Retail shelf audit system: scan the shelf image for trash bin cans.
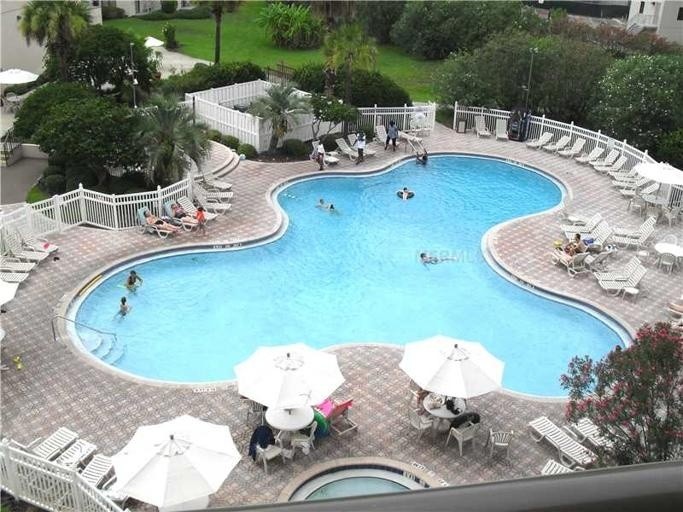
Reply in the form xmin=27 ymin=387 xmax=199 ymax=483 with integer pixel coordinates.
xmin=456 ymin=118 xmax=467 ymax=133
xmin=86 ymin=0 xmax=103 ymax=26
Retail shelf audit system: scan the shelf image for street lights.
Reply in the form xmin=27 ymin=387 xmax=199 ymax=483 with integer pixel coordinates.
xmin=524 ymin=47 xmax=539 ymax=111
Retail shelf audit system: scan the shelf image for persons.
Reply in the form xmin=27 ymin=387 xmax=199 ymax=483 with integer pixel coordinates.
xmin=125 ymin=270 xmax=144 ymax=296
xmin=192 ymin=206 xmax=209 ymax=235
xmin=314 ymin=137 xmax=326 ymax=170
xmin=354 ymin=129 xmax=367 ymax=164
xmin=143 ymin=209 xmax=178 ymax=233
xmin=169 ymin=202 xmax=197 ymax=224
xmin=383 ymin=121 xmax=399 ymax=151
xmin=421 ymin=253 xmax=458 ymax=270
xmin=316 ymin=199 xmax=337 ymax=212
xmin=114 ymin=297 xmax=132 ymax=320
xmin=398 ymin=186 xmax=415 ymax=200
xmin=569 ymin=234 xmax=587 ymax=257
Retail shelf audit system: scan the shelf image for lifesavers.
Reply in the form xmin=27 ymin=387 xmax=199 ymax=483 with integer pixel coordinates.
xmin=397 ymin=191 xmax=414 ymax=198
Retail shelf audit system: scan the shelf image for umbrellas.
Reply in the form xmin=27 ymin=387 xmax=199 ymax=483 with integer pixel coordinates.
xmin=109 ymin=413 xmax=242 ymax=508
xmin=233 ymin=342 xmax=348 ymax=413
xmin=144 ymin=35 xmax=168 ymax=50
xmin=633 ymin=159 xmax=683 ymax=201
xmin=0 ymin=68 xmax=41 ymax=86
xmin=399 ymin=335 xmax=509 ymax=402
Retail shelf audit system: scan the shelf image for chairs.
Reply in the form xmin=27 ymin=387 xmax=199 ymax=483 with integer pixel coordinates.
xmin=473 ymin=114 xmax=509 ymax=142
xmin=0 ymin=218 xmax=59 ymax=284
xmin=311 ymin=125 xmax=423 ymax=169
xmin=9 ymin=425 xmax=129 ymax=509
xmin=239 ymin=396 xmax=359 ymax=473
xmin=135 ymin=165 xmax=233 ymax=240
xmin=408 ymin=380 xmax=607 ymax=478
xmin=527 ymin=132 xmax=683 ymax=303
